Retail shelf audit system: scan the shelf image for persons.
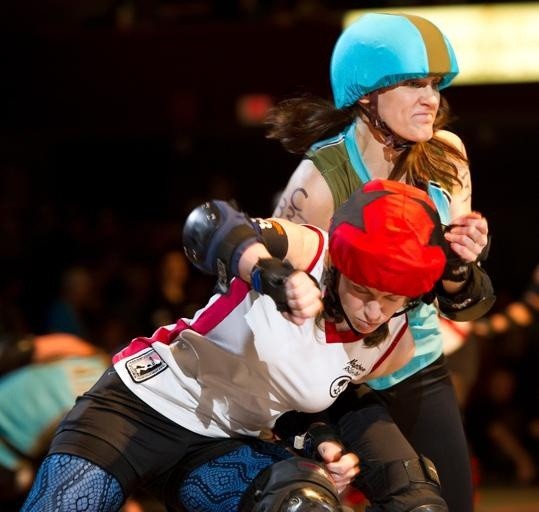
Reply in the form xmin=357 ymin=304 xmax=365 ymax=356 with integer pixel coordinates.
xmin=18 ymin=178 xmax=448 ymax=511
xmin=454 ymin=286 xmax=539 ymax=483
xmin=1 ymin=164 xmax=249 ymax=511
xmin=261 ymin=12 xmax=500 ymax=512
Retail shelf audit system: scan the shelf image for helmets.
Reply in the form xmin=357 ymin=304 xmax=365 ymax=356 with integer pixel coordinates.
xmin=328 ymin=179 xmax=448 ymax=298
xmin=329 ymin=13 xmax=459 ymax=113
